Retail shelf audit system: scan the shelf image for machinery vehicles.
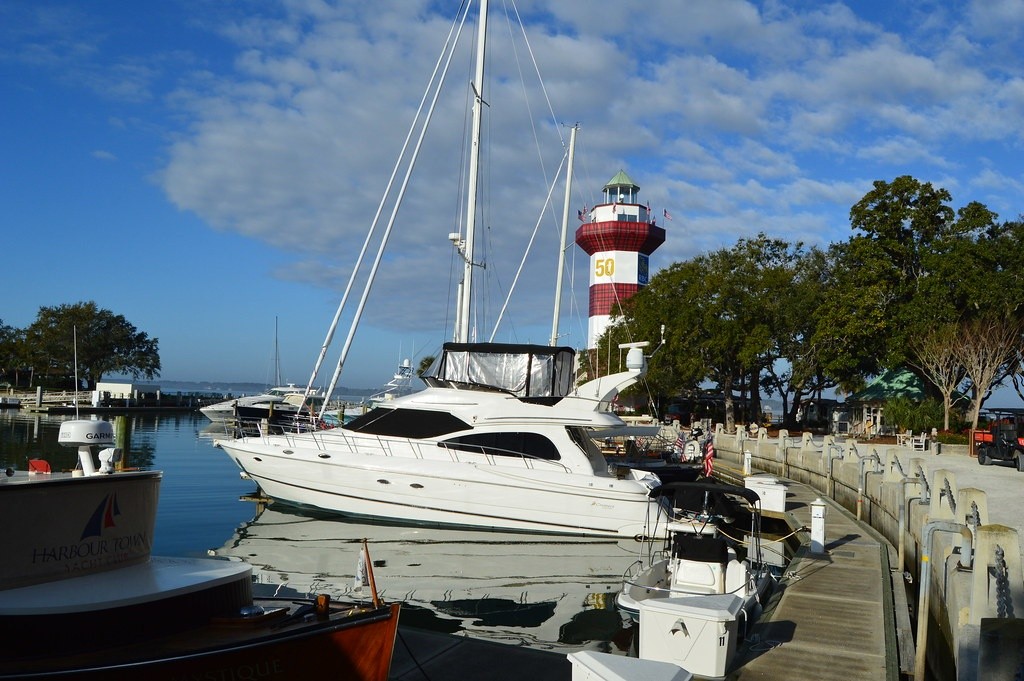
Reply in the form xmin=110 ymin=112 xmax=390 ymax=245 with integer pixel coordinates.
xmin=972 ymin=406 xmax=1024 ymax=471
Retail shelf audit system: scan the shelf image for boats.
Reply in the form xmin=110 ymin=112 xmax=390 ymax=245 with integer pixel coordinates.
xmin=598 ymin=480 xmax=792 ymax=631
xmin=0 ymin=405 xmax=400 ymax=679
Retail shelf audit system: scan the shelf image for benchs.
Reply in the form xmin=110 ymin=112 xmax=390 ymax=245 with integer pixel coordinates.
xmin=693 ymin=418 xmax=712 ymax=432
xmin=910 ymin=432 xmax=927 ymax=452
xmin=896 ymin=429 xmax=912 ymax=446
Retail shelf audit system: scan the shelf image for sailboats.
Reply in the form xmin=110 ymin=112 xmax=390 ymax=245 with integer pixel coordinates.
xmin=197 ymin=0 xmax=738 ymax=538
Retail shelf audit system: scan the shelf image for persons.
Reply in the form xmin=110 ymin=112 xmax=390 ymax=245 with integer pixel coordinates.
xmin=832 ymin=407 xmax=847 ymax=436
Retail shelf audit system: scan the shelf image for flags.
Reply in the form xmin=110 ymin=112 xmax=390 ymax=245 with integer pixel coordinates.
xmin=577 ymin=208 xmax=586 ymax=224
xmin=612 ymin=200 xmax=616 ymax=214
xmin=702 ymin=431 xmax=714 ymax=478
xmin=675 ymin=431 xmax=689 ymax=463
xmin=582 ymin=203 xmax=588 ymax=216
xmin=663 ymin=208 xmax=672 ymax=221
xmin=647 ymin=203 xmax=650 ymax=216
